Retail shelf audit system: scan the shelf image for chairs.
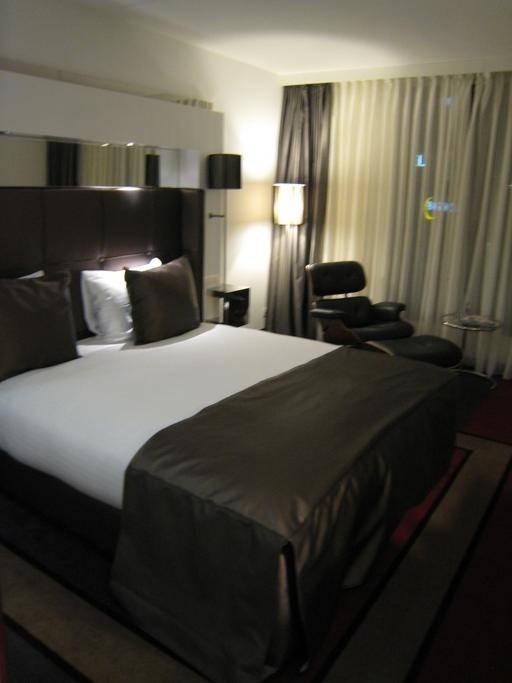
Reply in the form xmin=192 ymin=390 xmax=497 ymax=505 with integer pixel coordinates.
xmin=305 ymin=260 xmax=463 ymax=371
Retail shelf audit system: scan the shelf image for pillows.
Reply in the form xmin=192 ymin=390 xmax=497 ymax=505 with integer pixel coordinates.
xmin=0 ymin=271 xmax=83 ymax=383
xmin=124 ymin=254 xmax=202 ymax=345
xmin=16 ymin=267 xmax=45 ymax=277
xmin=80 ymin=256 xmax=164 ymax=339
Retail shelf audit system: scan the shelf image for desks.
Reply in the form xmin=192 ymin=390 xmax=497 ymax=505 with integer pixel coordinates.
xmin=439 ymin=312 xmax=502 ymax=404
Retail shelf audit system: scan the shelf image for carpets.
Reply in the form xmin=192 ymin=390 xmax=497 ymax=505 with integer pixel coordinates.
xmin=1 ymin=429 xmax=511 ymax=683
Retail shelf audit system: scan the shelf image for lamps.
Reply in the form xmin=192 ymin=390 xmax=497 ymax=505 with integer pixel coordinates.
xmin=272 ymin=183 xmax=305 ymax=226
xmin=1 ymin=185 xmax=460 ymax=683
xmin=205 ymin=154 xmax=242 ymax=323
